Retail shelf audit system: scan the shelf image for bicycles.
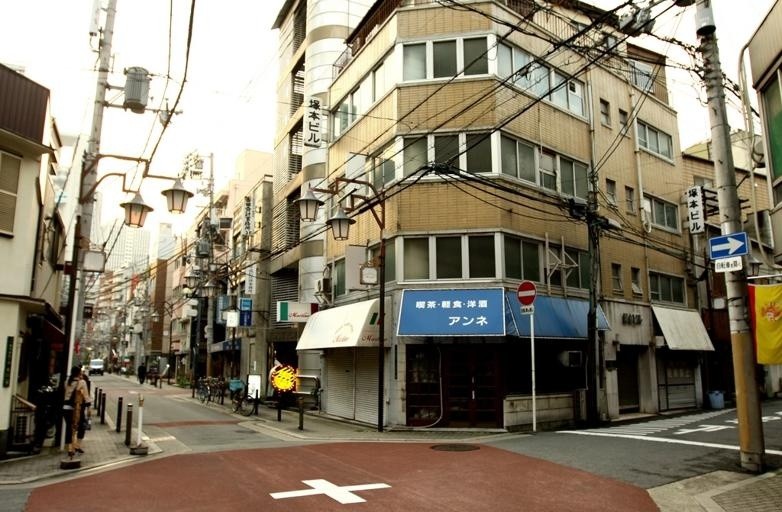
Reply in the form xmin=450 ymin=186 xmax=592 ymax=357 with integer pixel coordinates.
xmin=198 ymin=376 xmax=255 ymax=417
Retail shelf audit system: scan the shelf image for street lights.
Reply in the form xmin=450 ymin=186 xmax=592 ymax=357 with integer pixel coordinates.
xmin=53 ymin=151 xmax=195 ymax=453
xmin=292 ymin=176 xmax=386 ymax=432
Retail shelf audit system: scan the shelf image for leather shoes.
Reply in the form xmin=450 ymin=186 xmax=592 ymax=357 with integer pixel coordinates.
xmin=68 ymin=451 xmax=75 ymax=458
xmin=74 ymin=448 xmax=83 ymax=454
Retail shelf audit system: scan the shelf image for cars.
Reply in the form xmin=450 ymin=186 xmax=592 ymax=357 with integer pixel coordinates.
xmin=88 ymin=359 xmax=104 ymax=376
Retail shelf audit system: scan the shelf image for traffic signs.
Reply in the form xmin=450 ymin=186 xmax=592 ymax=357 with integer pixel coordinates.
xmin=518 ymin=280 xmax=538 ymax=306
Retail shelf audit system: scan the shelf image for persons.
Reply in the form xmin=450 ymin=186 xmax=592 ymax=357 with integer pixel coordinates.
xmin=138 ymin=362 xmax=147 ymax=384
xmin=63 ymin=364 xmax=92 ymax=458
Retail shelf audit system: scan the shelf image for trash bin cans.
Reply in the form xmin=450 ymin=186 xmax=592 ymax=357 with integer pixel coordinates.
xmin=574 ymin=389 xmax=594 ymax=428
xmin=11 ymin=407 xmax=35 ymax=451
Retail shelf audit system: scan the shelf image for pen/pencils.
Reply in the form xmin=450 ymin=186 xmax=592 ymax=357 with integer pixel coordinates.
xmin=708 ymin=391 xmax=725 ymax=409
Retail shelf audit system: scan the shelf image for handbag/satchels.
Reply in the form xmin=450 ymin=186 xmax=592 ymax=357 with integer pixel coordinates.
xmin=81 ymin=417 xmax=92 ymax=429
xmin=68 ymin=390 xmax=86 ymax=413
